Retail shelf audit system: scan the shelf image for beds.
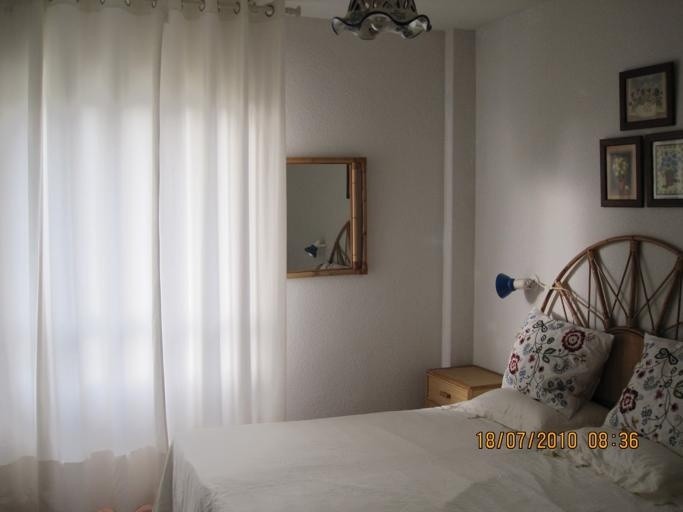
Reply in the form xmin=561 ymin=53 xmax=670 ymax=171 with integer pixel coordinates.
xmin=150 ymin=387 xmax=682 ymax=511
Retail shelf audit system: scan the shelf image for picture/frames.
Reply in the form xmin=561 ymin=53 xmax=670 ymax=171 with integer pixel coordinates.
xmin=598 ymin=60 xmax=683 ymax=207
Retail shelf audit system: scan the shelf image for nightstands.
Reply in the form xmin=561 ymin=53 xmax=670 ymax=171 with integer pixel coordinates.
xmin=423 ymin=365 xmax=504 ymax=410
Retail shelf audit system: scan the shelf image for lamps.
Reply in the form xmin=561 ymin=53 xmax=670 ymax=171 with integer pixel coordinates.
xmin=495 ymin=273 xmax=537 ymax=299
xmin=329 ymin=1 xmax=432 ymax=40
xmin=303 ymin=239 xmax=327 ymax=259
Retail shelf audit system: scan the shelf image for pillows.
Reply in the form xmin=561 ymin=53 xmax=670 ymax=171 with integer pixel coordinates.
xmin=502 ymin=307 xmax=616 ymax=420
xmin=457 ymin=386 xmax=610 ymax=441
xmin=601 ymin=332 xmax=682 ymax=456
xmin=538 ymin=427 xmax=682 ymax=504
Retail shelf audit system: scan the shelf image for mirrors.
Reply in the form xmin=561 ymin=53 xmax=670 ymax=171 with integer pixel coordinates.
xmin=284 ymin=157 xmax=368 ymax=279
